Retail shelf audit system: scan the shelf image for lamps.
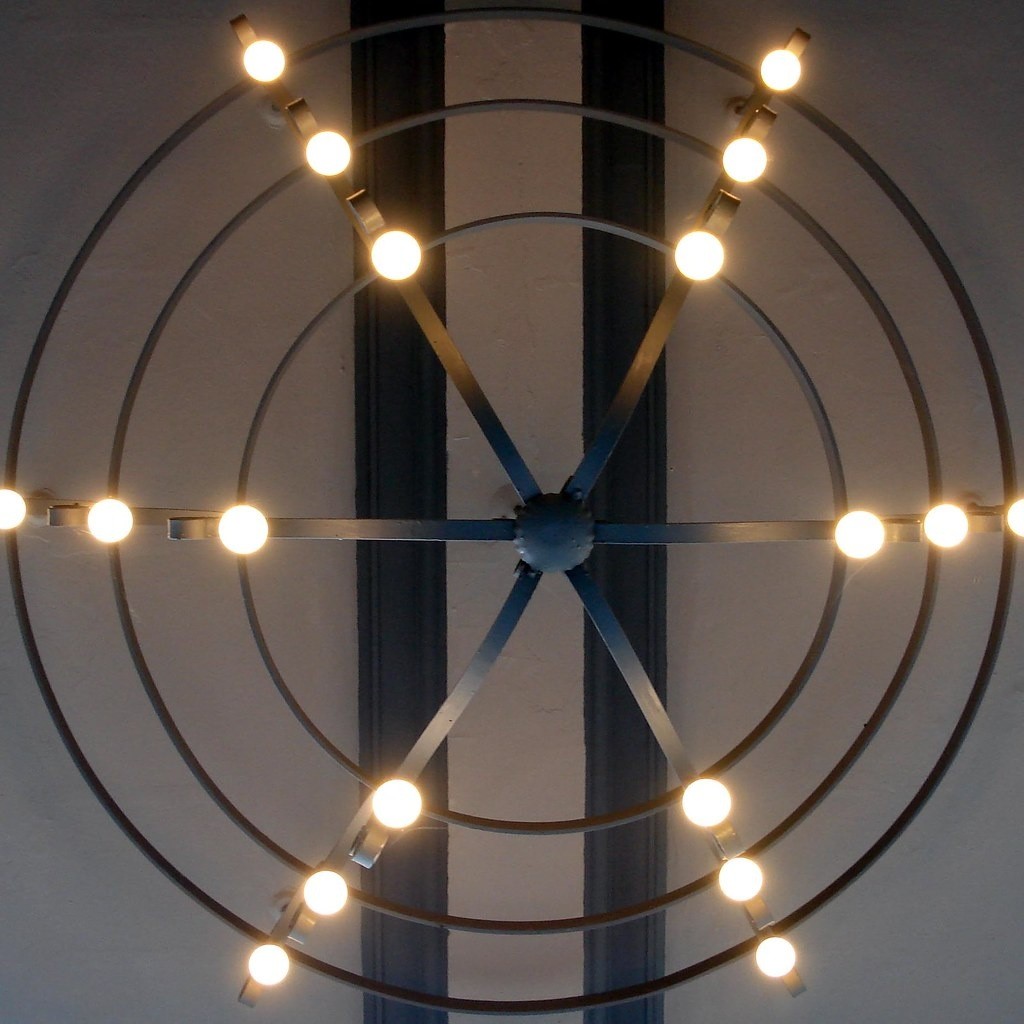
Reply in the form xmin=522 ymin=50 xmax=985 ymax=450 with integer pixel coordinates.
xmin=0 ymin=10 xmax=1024 ymax=1015
